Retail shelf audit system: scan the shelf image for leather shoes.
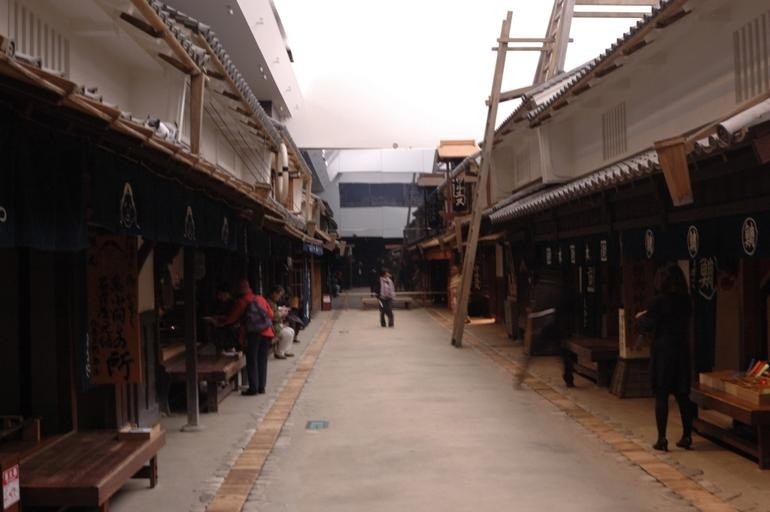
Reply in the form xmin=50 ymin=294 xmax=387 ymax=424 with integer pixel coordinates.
xmin=242 ymin=390 xmax=258 ymax=395
xmin=259 ymin=388 xmax=264 ymax=393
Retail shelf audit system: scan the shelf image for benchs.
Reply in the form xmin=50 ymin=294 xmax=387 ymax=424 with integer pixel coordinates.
xmin=559 ymin=335 xmax=618 ymax=387
xmin=165 ymin=352 xmax=247 ymax=414
xmin=20 ymin=428 xmax=166 ymax=511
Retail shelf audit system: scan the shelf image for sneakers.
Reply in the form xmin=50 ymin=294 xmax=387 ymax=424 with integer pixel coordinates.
xmin=275 ymin=353 xmax=286 ymax=359
xmin=564 ymin=375 xmax=575 ymax=387
xmin=285 ymin=353 xmax=294 ymax=356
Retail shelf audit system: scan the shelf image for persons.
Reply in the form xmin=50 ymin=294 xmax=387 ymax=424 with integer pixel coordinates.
xmin=635 ymin=268 xmax=696 ymax=452
xmin=375 ymin=268 xmax=396 ymax=327
xmin=366 ymin=265 xmax=378 ymax=296
xmin=205 ymin=275 xmax=302 ymax=397
xmin=334 ymin=272 xmax=345 ymax=296
xmin=448 ymin=265 xmax=472 ymax=324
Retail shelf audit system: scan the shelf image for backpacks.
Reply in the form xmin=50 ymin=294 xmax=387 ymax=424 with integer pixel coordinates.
xmin=240 ymin=294 xmax=273 ymax=334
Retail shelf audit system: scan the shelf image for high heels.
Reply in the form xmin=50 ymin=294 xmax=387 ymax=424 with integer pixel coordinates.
xmin=654 ymin=437 xmax=667 ymax=451
xmin=677 ymin=435 xmax=691 ymax=449
